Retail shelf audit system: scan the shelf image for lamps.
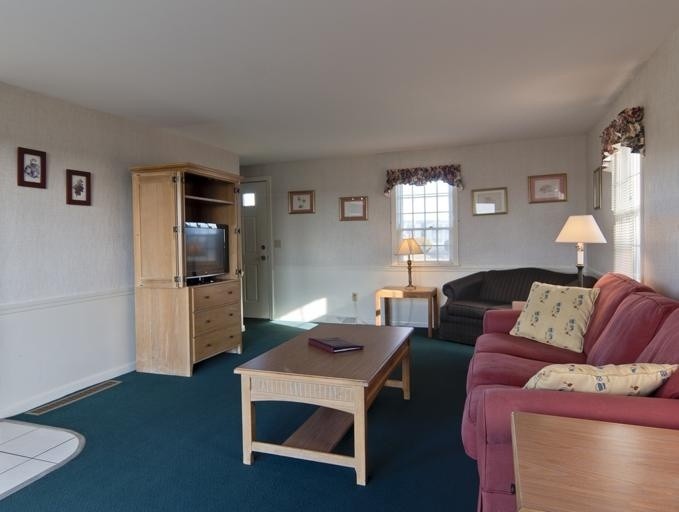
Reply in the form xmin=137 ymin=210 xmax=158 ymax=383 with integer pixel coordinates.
xmin=553 ymin=214 xmax=608 ymax=287
xmin=394 ymin=238 xmax=424 ymax=290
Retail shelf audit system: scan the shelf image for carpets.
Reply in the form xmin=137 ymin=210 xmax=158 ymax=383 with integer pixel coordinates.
xmin=0 ymin=418 xmax=87 ymax=505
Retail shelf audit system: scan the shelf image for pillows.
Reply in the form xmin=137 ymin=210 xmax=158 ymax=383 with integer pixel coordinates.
xmin=508 ymin=281 xmax=600 ymax=353
xmin=522 ymin=363 xmax=679 ymax=396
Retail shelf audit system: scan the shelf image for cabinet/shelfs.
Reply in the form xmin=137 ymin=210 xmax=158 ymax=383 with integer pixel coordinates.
xmin=128 ymin=162 xmax=244 ymax=378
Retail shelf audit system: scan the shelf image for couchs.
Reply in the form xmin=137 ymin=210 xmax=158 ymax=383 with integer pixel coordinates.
xmin=461 ymin=272 xmax=678 ymax=511
xmin=439 ymin=268 xmax=598 ymax=346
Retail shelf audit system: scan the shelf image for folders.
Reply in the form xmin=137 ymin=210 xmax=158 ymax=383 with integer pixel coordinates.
xmin=308 ymin=337 xmax=363 ymax=353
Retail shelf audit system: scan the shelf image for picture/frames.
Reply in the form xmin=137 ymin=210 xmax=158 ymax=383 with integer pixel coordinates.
xmin=339 ymin=195 xmax=369 ymax=222
xmin=592 ymin=165 xmax=603 ymax=209
xmin=15 ymin=147 xmax=48 ymax=189
xmin=527 ymin=172 xmax=568 ymax=204
xmin=65 ymin=169 xmax=93 ymax=206
xmin=287 ymin=189 xmax=316 ymax=215
xmin=470 ymin=186 xmax=510 ymax=217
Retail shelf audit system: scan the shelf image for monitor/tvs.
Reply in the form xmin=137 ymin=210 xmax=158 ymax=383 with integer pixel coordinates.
xmin=184 ymin=222 xmax=230 ymax=285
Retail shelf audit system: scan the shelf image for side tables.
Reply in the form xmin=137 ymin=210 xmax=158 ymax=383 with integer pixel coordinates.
xmin=374 ymin=286 xmax=438 ymax=338
xmin=508 ymin=409 xmax=679 ymax=510
